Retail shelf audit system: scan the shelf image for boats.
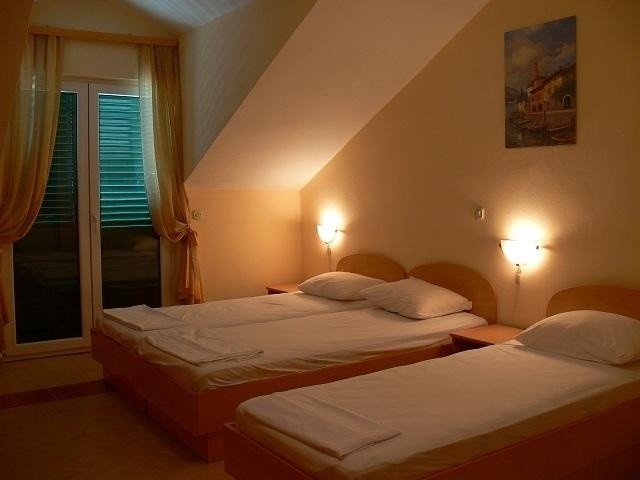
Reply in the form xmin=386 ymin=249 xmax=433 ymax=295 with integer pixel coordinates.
xmin=510 ymin=110 xmax=577 ymax=142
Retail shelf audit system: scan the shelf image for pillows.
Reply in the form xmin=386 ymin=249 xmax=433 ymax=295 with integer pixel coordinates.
xmin=514 ymin=310 xmax=640 ymax=364
xmin=295 ymin=271 xmax=388 ymax=301
xmin=361 ymin=276 xmax=472 ymax=320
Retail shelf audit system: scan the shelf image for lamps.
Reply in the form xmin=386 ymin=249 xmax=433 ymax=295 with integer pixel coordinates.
xmin=316 ymin=224 xmax=338 ymax=254
xmin=498 ymin=238 xmax=539 ymax=285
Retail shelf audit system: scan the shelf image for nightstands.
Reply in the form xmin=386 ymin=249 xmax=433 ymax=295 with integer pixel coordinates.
xmin=266 ymin=283 xmax=301 ymax=294
xmin=449 ymin=324 xmax=524 ymax=354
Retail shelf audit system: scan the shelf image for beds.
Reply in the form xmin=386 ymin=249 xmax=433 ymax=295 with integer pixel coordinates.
xmin=89 ymin=254 xmax=498 ymax=463
xmin=224 ymin=287 xmax=640 ymax=480
